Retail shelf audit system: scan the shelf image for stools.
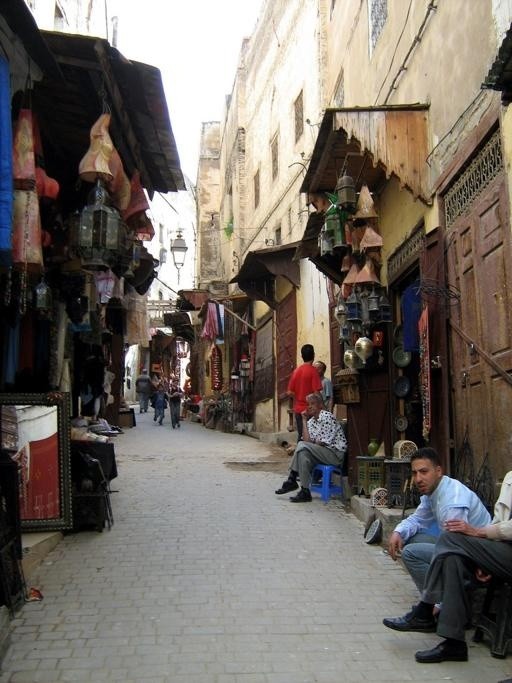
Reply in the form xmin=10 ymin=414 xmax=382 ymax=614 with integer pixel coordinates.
xmin=310 ymin=464 xmax=345 ymax=501
xmin=472 ymin=577 xmax=512 ymax=657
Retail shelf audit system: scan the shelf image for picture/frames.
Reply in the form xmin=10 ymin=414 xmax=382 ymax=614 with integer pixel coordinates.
xmin=0 ymin=392 xmax=74 ymax=531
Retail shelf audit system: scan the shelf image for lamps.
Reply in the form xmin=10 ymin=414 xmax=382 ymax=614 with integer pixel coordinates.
xmin=336 ymin=160 xmax=357 ymax=213
xmin=158 ymin=223 xmax=167 ymax=263
xmin=170 ymin=230 xmax=189 ymax=285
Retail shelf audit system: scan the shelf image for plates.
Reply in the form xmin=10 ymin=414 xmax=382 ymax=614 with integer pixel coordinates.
xmin=393 ymin=414 xmax=408 ymax=432
xmin=392 ymin=376 xmax=410 ymax=397
xmin=393 ymin=322 xmax=408 ymax=348
xmin=392 ymin=346 xmax=412 ymax=367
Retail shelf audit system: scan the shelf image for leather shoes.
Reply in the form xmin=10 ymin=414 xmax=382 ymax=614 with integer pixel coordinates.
xmin=275 ymin=482 xmax=299 ymax=494
xmin=415 ymin=642 xmax=467 ymax=663
xmin=383 ymin=610 xmax=435 ymax=632
xmin=289 ymin=490 xmax=312 ymax=503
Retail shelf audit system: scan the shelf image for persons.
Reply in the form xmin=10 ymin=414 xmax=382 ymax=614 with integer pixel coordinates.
xmin=135 ymin=369 xmax=156 ymax=413
xmin=382 ymin=469 xmax=511 ymax=665
xmin=275 ymin=394 xmax=348 ymax=504
xmin=149 ymin=384 xmax=169 ymax=425
xmin=386 ymin=447 xmax=491 ymax=615
xmin=313 ymin=360 xmax=335 ymax=413
xmin=286 ymin=344 xmax=328 ymax=444
xmin=168 ymin=377 xmax=184 ymax=428
